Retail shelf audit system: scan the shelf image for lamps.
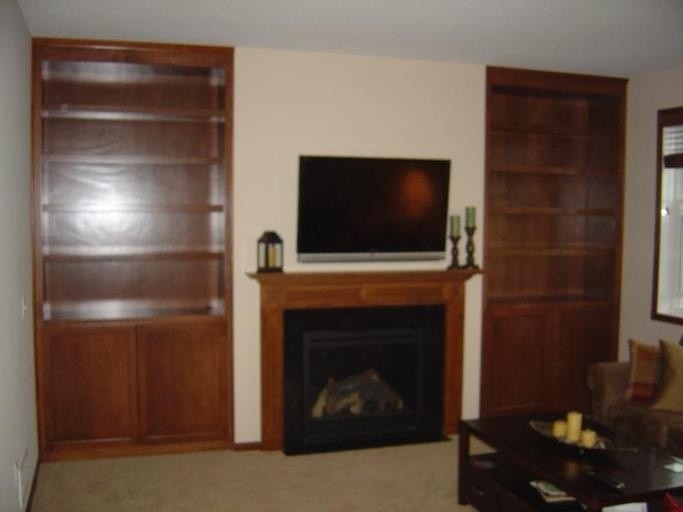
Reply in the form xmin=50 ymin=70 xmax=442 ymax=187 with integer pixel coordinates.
xmin=256 ymin=231 xmax=284 ymax=273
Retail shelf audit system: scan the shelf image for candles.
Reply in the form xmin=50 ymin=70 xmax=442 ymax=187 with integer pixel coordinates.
xmin=450 ymin=203 xmax=477 ymax=239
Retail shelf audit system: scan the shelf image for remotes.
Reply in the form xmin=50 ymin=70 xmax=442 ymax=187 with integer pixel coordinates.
xmin=589 ymin=470 xmax=625 ymax=491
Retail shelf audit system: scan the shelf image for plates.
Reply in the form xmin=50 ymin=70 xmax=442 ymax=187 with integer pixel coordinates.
xmin=529 ymin=420 xmax=639 ymax=455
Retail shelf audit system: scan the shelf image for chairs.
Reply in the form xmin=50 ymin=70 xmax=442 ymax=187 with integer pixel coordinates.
xmin=583 ymin=334 xmax=682 ymax=455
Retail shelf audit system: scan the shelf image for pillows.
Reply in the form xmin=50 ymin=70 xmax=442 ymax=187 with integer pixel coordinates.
xmin=624 ymin=338 xmax=683 ymax=411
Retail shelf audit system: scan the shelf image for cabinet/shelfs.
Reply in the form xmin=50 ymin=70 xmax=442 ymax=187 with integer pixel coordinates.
xmin=477 ymin=65 xmax=629 ymax=420
xmin=31 ymin=37 xmax=234 ymax=466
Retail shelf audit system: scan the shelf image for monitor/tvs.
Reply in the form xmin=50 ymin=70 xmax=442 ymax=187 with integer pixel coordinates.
xmin=296 ymin=154 xmax=452 ymax=264
